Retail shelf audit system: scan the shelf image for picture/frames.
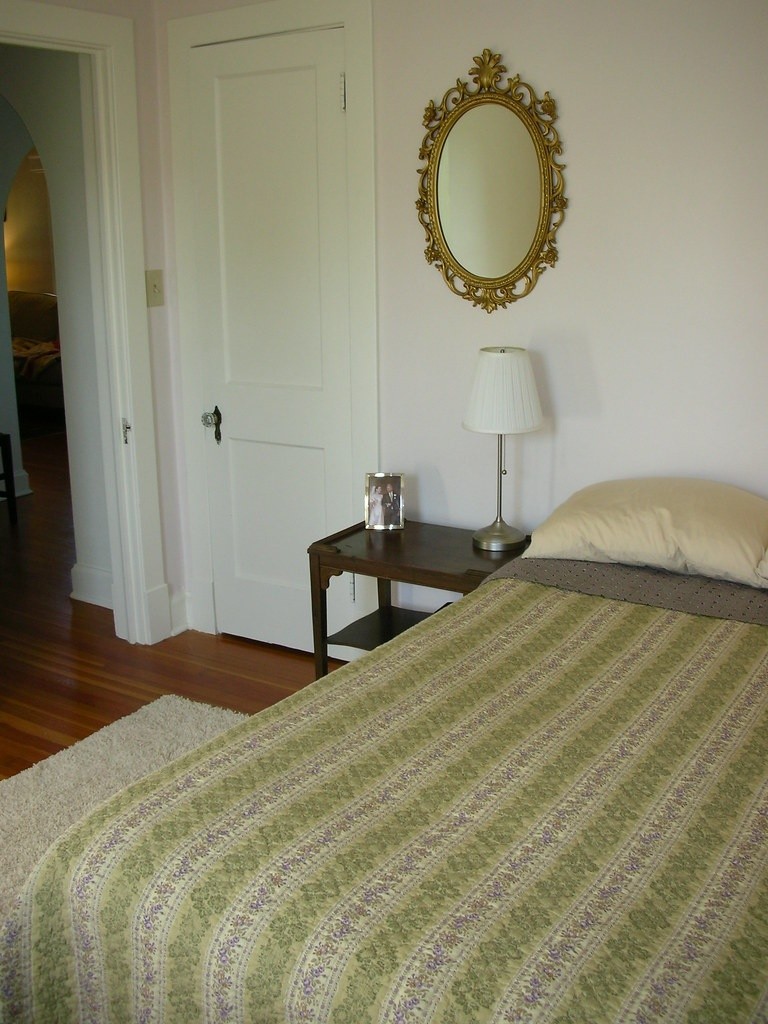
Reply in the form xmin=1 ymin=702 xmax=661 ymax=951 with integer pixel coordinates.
xmin=363 ymin=473 xmax=405 ymax=530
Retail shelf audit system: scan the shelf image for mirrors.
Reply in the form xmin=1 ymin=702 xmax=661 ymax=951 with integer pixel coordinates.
xmin=416 ymin=50 xmax=568 ymax=315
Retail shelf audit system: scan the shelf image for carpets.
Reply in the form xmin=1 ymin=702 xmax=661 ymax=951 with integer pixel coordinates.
xmin=0 ymin=694 xmax=261 ymax=916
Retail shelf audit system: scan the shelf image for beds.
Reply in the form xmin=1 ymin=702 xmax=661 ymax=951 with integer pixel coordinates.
xmin=30 ymin=550 xmax=768 ymax=1024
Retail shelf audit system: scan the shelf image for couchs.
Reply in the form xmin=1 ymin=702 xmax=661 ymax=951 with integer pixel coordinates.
xmin=9 ymin=289 xmax=65 ymax=426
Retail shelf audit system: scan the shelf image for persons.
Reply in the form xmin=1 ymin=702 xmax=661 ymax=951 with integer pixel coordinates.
xmin=369 ymin=484 xmax=400 ymax=525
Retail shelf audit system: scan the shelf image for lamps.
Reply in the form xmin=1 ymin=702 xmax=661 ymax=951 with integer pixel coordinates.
xmin=463 ymin=345 xmax=542 ymax=552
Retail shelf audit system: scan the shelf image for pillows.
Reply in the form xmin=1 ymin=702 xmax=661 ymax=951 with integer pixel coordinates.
xmin=524 ymin=480 xmax=768 ymax=590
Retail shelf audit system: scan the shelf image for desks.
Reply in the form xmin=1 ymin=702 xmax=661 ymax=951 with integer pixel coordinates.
xmin=0 ymin=433 xmax=18 ymax=526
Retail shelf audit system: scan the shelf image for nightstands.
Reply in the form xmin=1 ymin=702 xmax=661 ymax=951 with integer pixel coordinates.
xmin=307 ymin=518 xmax=531 ymax=681
